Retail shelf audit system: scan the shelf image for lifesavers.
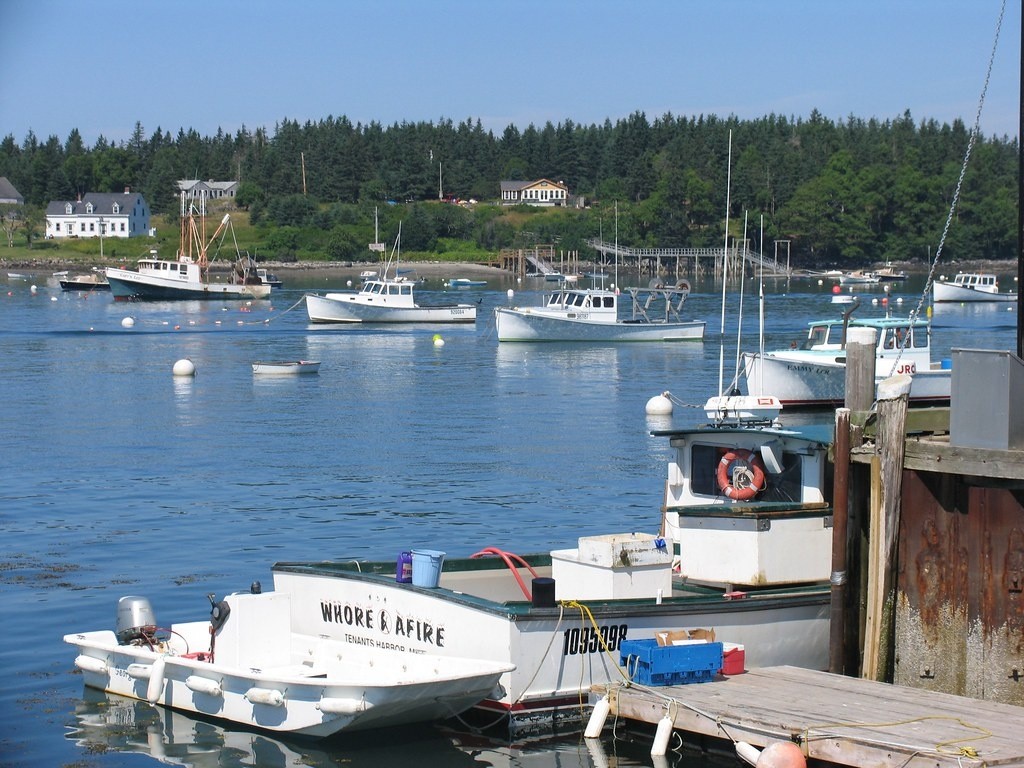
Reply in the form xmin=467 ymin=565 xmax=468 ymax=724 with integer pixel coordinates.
xmin=717 ymin=449 xmax=764 ymax=500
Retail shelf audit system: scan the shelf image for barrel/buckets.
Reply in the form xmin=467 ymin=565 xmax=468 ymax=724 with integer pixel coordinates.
xmin=396 ymin=551 xmax=412 ymax=584
xmin=411 ymin=549 xmax=447 ymax=589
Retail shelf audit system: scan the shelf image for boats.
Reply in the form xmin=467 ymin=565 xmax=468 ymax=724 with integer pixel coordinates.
xmin=544 ymin=270 xmax=565 ymax=282
xmin=7 ymin=273 xmax=25 ymax=277
xmin=494 ymin=199 xmax=706 ymax=343
xmin=59 ymin=271 xmax=111 ymax=291
xmin=61 ymin=595 xmax=518 ymax=740
xmin=870 ymin=256 xmax=909 ymax=281
xmin=257 ymin=268 xmax=283 ymax=290
xmin=306 ymin=220 xmax=477 ymax=324
xmin=53 ymin=271 xmax=68 ymax=276
xmin=272 ymin=129 xmax=951 ymax=746
xmin=744 ymin=214 xmax=952 ymax=414
xmin=252 ymin=360 xmax=322 ymax=374
xmin=449 ymin=278 xmax=488 ymax=285
xmin=839 ymin=270 xmax=882 ymax=284
xmin=933 ymin=273 xmax=1018 ymax=303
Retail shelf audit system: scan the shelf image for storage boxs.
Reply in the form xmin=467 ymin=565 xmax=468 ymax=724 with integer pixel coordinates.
xmin=578 ymin=532 xmax=674 ymax=569
xmin=716 ymin=649 xmax=745 ymax=676
xmin=619 ymin=638 xmax=723 ymax=688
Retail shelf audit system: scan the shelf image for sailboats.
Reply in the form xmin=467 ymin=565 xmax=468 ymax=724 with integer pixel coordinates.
xmin=104 ymin=190 xmax=271 ymax=302
